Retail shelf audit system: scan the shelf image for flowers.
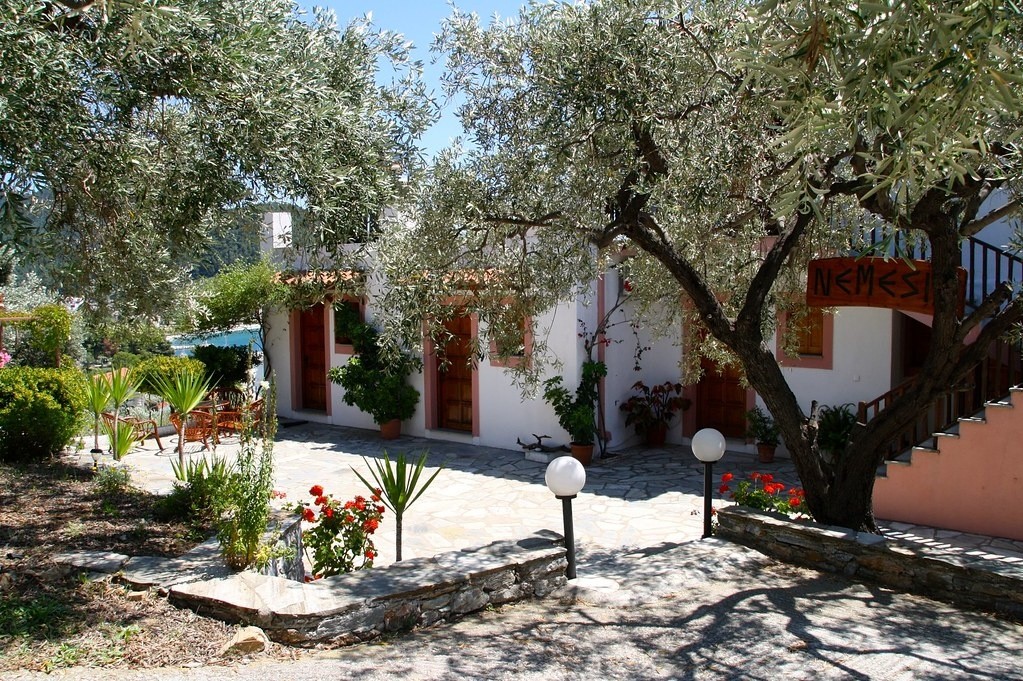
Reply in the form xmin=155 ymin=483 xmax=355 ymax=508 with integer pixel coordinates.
xmin=300 ymin=481 xmax=386 ymax=581
xmin=711 ymin=473 xmax=814 ymax=534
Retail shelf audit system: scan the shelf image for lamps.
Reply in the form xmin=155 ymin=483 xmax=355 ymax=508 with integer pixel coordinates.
xmin=691 ymin=428 xmax=727 ymax=462
xmin=544 ymin=455 xmax=588 ymax=496
xmin=90 ymin=448 xmax=104 ymax=462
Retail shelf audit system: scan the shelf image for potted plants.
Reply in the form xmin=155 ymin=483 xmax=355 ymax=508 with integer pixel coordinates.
xmin=817 ymin=400 xmax=856 ymax=461
xmin=543 ymin=360 xmax=606 ymax=465
xmin=517 ymin=434 xmax=569 ymax=463
xmin=325 ymin=323 xmax=425 ymax=440
xmin=619 ymin=379 xmax=691 ymax=451
xmin=743 ymin=405 xmax=783 ymax=464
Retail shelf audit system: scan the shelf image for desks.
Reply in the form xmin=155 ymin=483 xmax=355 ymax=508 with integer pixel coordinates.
xmin=175 ymin=400 xmax=229 ymax=451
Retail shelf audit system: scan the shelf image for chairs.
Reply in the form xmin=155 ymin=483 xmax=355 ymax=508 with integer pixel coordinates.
xmin=101 ymin=412 xmax=164 ymax=454
xmin=172 ymin=408 xmax=219 ymax=454
xmin=216 ymin=398 xmax=267 ymax=444
